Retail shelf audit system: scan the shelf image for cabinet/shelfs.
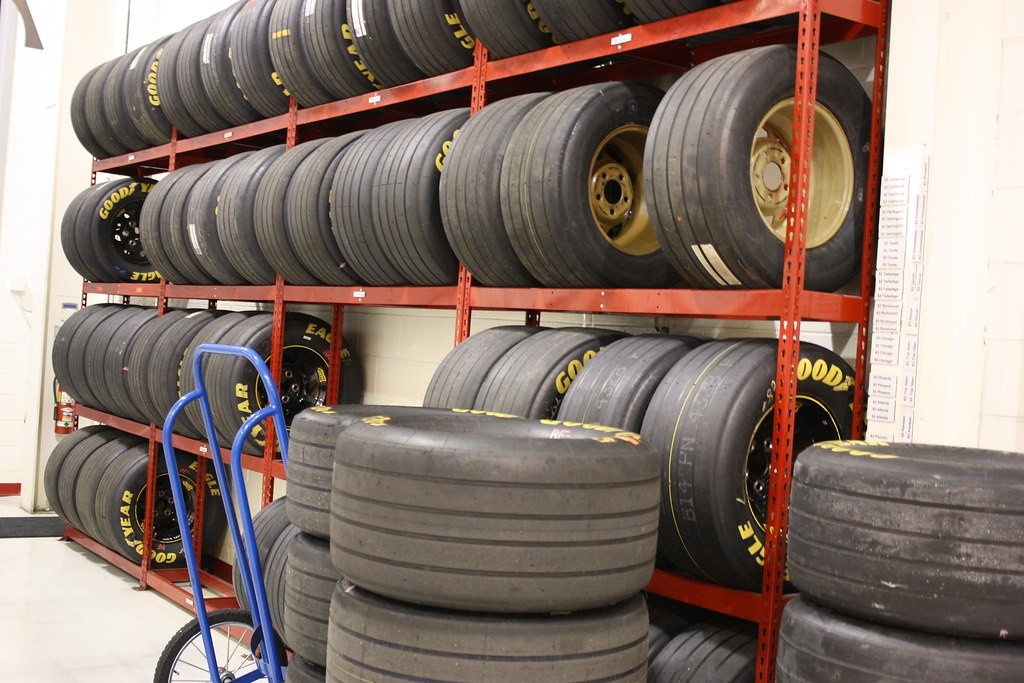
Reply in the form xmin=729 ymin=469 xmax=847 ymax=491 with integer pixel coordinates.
xmin=62 ymin=0 xmax=891 ymax=683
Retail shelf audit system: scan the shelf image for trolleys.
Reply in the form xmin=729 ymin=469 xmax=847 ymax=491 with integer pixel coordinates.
xmin=152 ymin=344 xmax=288 ymax=683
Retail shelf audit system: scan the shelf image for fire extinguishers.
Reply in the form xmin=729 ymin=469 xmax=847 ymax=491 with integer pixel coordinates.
xmin=53 ymin=377 xmax=76 ymax=434
